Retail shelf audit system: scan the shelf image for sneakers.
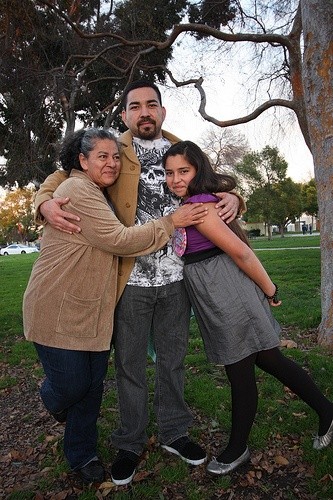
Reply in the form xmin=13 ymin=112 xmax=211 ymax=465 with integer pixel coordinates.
xmin=161 ymin=435 xmax=208 ymax=464
xmin=110 ymin=448 xmax=146 ymax=485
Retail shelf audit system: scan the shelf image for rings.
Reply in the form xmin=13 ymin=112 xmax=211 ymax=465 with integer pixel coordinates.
xmin=231 ymin=209 xmax=235 ymax=213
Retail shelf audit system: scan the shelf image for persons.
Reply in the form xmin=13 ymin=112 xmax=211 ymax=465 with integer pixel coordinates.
xmin=23 ymin=128 xmax=208 ymax=483
xmin=302 ymin=224 xmax=313 ymax=234
xmin=34 ymin=80 xmax=247 ymax=484
xmin=161 ymin=141 xmax=333 ymax=475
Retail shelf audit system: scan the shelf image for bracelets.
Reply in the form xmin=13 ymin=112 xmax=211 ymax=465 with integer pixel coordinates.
xmin=264 ymin=283 xmax=279 ymax=299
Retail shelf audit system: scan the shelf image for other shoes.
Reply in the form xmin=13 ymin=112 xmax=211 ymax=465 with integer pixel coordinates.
xmin=53 ymin=409 xmax=66 ymax=423
xmin=207 ymin=444 xmax=251 ymax=476
xmin=311 ymin=420 xmax=333 ymax=449
xmin=77 ymin=460 xmax=106 ymax=483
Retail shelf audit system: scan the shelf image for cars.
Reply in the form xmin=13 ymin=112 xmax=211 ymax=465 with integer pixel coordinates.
xmin=0 ymin=243 xmax=38 ymax=256
